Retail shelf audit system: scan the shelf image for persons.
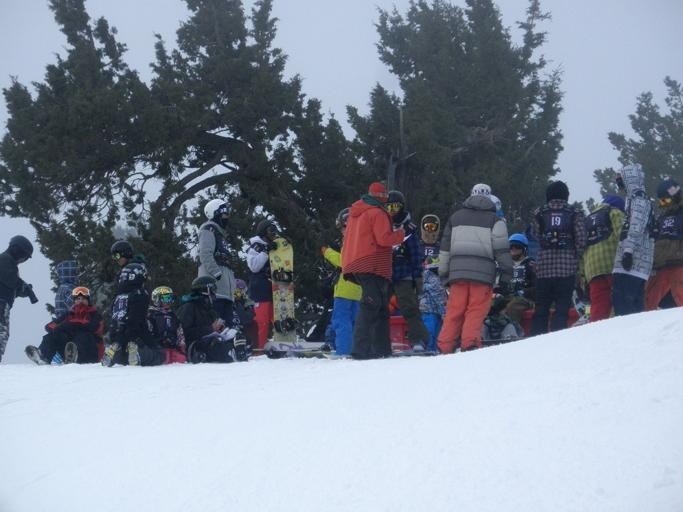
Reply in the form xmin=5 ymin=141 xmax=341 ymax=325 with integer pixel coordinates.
xmin=1 ymin=165 xmax=683 ymax=367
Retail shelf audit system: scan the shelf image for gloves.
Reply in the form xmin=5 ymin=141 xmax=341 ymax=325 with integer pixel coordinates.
xmin=46 ymin=303 xmax=56 ymax=313
xmin=320 ymin=245 xmax=328 ymax=256
xmin=265 ymin=242 xmax=278 ymax=252
xmin=498 ymin=270 xmax=513 ymax=283
xmin=621 ymin=251 xmax=633 ymax=272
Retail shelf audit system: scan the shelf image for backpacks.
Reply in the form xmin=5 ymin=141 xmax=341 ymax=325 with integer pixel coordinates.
xmin=584 ymin=207 xmax=613 ymax=243
xmin=539 ymin=204 xmax=572 ymax=251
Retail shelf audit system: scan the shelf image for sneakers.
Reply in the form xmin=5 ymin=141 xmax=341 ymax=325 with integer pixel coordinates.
xmin=101 ymin=341 xmax=121 ymax=367
xmin=25 ymin=344 xmax=54 ymax=364
xmin=320 ymin=339 xmax=339 ymax=353
xmin=127 ymin=341 xmax=143 ymax=366
xmin=63 ymin=341 xmax=79 ymax=364
xmin=410 ymin=343 xmax=427 ymax=353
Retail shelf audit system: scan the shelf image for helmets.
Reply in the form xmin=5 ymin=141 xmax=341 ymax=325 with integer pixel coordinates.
xmin=73 ymin=287 xmax=92 ymax=306
xmin=191 ymin=276 xmax=217 ymax=298
xmin=656 ymin=178 xmax=679 ymax=199
xmin=508 ymin=233 xmax=528 ymax=249
xmin=336 ymin=208 xmax=352 ymax=231
xmin=257 ymin=219 xmax=274 ymax=234
xmin=119 ymin=264 xmax=148 ymax=287
xmin=204 ymin=198 xmax=232 ymax=221
xmin=111 ymin=240 xmax=134 ymax=258
xmin=386 ymin=190 xmax=406 ymax=207
xmin=422 ymin=215 xmax=439 ymax=226
xmin=487 ymin=192 xmax=502 ymax=211
xmin=9 ymin=235 xmax=34 ymax=259
xmin=368 ymin=182 xmax=389 ymax=204
xmin=471 ymin=183 xmax=491 ymax=201
xmin=152 ymin=285 xmax=175 ymax=306
xmin=232 ymin=278 xmax=248 ymax=294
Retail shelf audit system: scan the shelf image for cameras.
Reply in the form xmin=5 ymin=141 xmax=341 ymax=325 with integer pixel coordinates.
xmin=22 ymin=284 xmax=38 ymax=304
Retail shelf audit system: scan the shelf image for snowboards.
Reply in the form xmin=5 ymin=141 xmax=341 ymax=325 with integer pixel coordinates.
xmin=264 ymin=342 xmax=335 ymax=358
xmin=269 ymin=238 xmax=296 ymax=342
xmin=479 ymin=339 xmax=529 ymax=342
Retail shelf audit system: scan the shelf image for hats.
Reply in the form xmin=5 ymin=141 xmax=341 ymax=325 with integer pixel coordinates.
xmin=546 ymin=181 xmax=569 ymax=201
